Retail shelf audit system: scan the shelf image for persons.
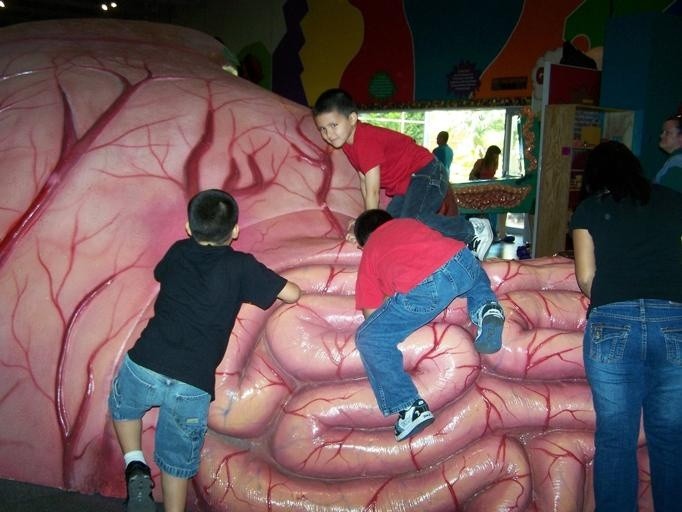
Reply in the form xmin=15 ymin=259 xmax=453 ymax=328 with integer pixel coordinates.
xmin=353 ymin=208 xmax=507 ymax=442
xmin=106 ymin=186 xmax=303 ymax=512
xmin=312 ymin=87 xmax=495 ymax=263
xmin=468 ymin=145 xmax=515 ymax=243
xmin=569 ymin=139 xmax=682 ymax=512
xmin=432 ymin=131 xmax=453 ymax=169
xmin=652 ymin=115 xmax=682 ymax=193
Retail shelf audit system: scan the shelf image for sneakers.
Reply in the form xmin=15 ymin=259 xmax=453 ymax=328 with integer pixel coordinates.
xmin=125 ymin=461 xmax=157 ymax=512
xmin=395 ymin=399 xmax=434 ymax=441
xmin=466 ymin=217 xmax=493 ymax=261
xmin=474 ymin=304 xmax=505 ymax=354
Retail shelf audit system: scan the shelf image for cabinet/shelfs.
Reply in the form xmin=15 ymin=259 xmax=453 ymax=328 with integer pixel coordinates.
xmin=534 ymin=104 xmax=634 ymax=258
